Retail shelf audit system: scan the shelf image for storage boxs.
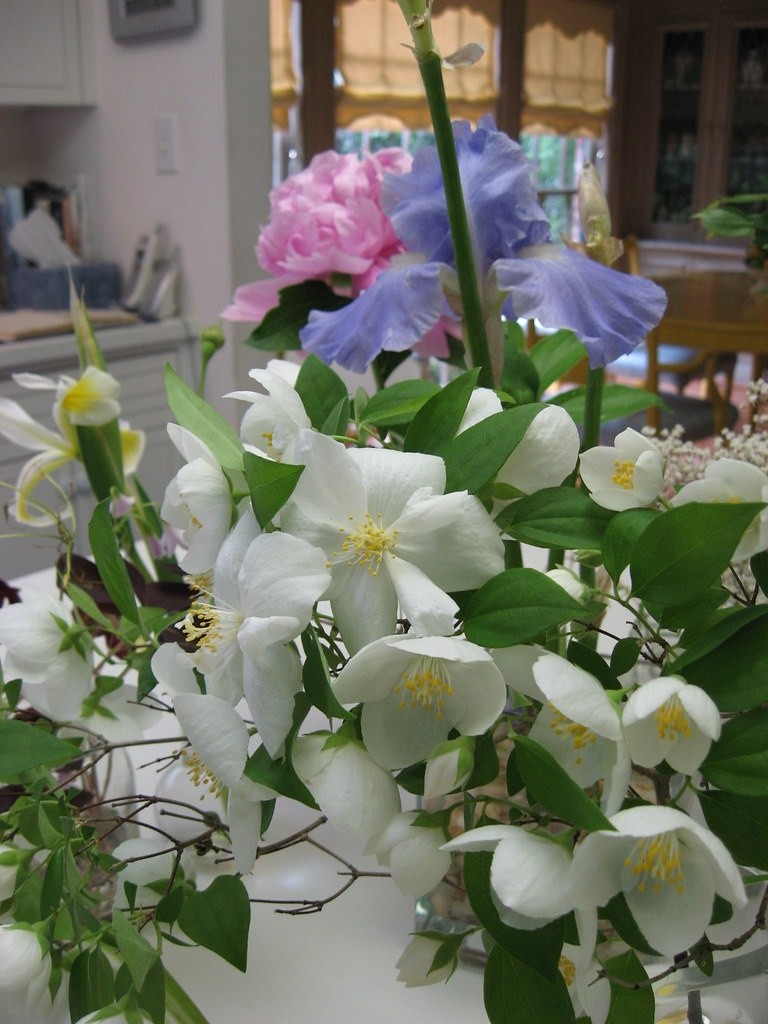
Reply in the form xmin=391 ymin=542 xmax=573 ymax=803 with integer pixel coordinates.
xmin=7 ymin=263 xmax=121 ymax=309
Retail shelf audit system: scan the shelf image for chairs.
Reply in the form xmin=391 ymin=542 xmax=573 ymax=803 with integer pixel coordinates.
xmin=523 ymin=236 xmax=737 ymax=452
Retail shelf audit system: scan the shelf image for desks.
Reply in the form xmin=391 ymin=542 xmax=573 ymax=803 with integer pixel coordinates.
xmin=1 ymin=533 xmax=768 ymax=1024
xmin=646 ymin=269 xmax=768 ymax=431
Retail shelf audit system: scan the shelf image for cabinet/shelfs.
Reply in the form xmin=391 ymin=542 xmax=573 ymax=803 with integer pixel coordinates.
xmin=625 ymin=0 xmax=768 ymax=243
xmin=0 ymin=0 xmax=98 ymax=105
xmin=0 ymin=322 xmax=196 ymax=581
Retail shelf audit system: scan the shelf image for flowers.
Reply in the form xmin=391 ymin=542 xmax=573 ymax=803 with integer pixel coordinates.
xmin=0 ymin=0 xmax=768 ymax=1024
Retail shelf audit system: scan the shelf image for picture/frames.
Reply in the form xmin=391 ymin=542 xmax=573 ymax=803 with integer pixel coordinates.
xmin=105 ymin=0 xmax=198 ymax=39
